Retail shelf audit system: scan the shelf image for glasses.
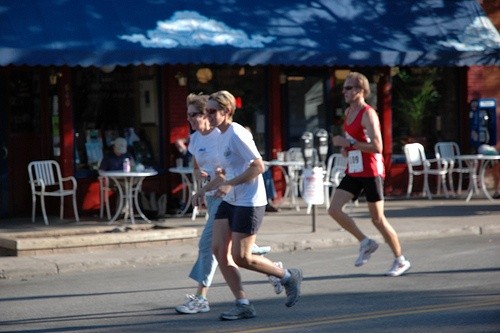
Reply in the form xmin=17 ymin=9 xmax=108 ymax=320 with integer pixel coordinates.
xmin=342 ymin=84 xmax=357 ymax=92
xmin=186 ymin=111 xmax=201 ymax=118
xmin=204 ymin=108 xmax=221 ymax=114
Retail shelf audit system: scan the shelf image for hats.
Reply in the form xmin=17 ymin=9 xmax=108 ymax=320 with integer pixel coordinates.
xmin=114 ymin=138 xmax=127 ymax=154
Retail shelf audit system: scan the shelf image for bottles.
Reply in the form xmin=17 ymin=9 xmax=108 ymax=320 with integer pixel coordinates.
xmin=122 ymin=157 xmax=131 ymax=173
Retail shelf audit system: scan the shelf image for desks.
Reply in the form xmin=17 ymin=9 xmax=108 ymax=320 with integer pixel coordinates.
xmin=99 ymin=169 xmax=158 ymax=224
xmin=264 ymin=161 xmax=304 ymax=211
xmin=452 ymin=154 xmax=500 ymax=203
xmin=168 ymin=167 xmax=209 ymax=220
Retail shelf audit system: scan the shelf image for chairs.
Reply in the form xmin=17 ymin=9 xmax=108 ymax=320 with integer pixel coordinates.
xmin=286 ymin=148 xmax=326 ymax=203
xmin=28 ymin=160 xmax=80 ymax=225
xmin=97 ymin=156 xmax=141 ymax=220
xmin=403 ymin=142 xmax=470 ymax=200
xmin=306 ymin=154 xmax=359 ymax=215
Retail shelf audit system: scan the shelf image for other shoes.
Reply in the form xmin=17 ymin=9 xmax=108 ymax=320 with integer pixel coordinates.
xmin=266 ymin=202 xmax=280 ymax=212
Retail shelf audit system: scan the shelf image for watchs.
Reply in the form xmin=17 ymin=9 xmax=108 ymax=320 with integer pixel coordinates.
xmin=348 ymin=139 xmax=355 ymax=149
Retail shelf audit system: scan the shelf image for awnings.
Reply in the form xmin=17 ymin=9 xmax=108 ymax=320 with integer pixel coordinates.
xmin=0 ymin=0 xmax=500 ymax=68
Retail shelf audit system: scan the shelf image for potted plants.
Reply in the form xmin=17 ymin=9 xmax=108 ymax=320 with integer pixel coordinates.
xmin=390 ymin=66 xmax=443 ymax=154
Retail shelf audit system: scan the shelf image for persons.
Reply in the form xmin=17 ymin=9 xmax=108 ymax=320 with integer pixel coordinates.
xmin=175 ymin=89 xmax=302 ymax=321
xmin=329 ymin=72 xmax=411 ymax=276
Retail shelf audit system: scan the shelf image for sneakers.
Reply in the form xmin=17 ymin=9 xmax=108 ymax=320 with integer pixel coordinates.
xmin=220 ymin=303 xmax=257 ymax=320
xmin=387 ymin=259 xmax=412 ymax=277
xmin=176 ymin=294 xmax=210 ymax=314
xmin=267 ymin=261 xmax=283 ymax=294
xmin=281 ymin=269 xmax=304 ymax=309
xmin=354 ymin=238 xmax=379 ymax=267
